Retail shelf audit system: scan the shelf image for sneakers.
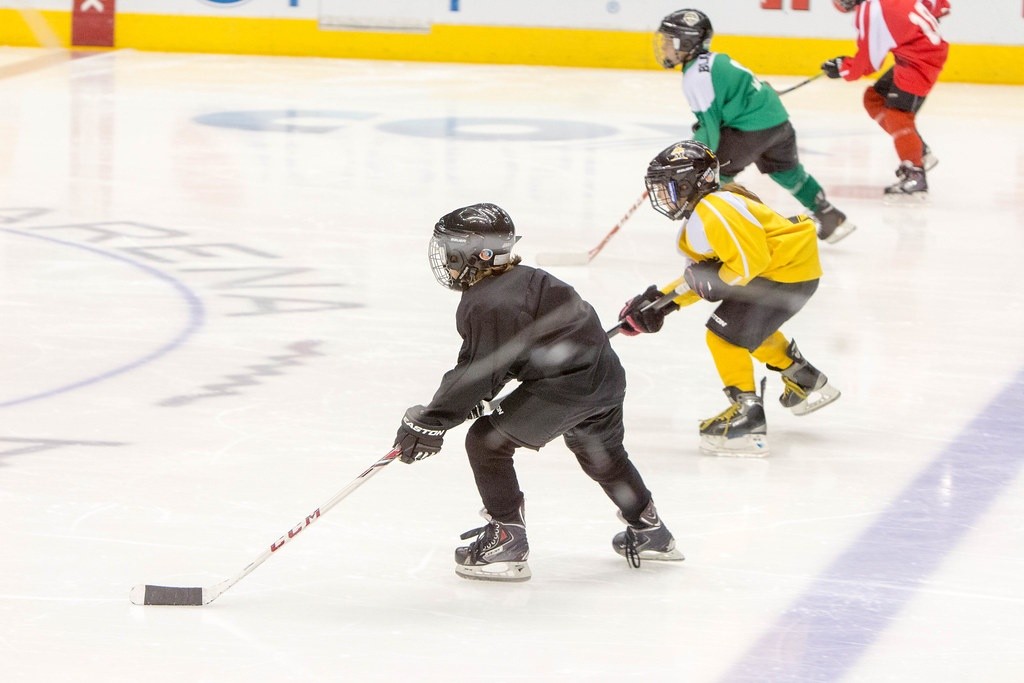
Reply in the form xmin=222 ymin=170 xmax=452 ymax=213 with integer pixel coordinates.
xmin=611 ymin=497 xmax=685 ymax=569
xmin=765 ymin=337 xmax=841 ymax=417
xmin=920 ymin=135 xmax=941 ymax=177
xmin=811 ymin=188 xmax=857 ymax=245
xmin=455 ymin=498 xmax=531 ymax=582
xmin=698 ymin=376 xmax=770 ymax=458
xmin=883 ymin=160 xmax=931 ymax=208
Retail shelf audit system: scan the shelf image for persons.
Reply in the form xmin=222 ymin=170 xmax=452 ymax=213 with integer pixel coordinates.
xmin=618 ymin=140 xmax=840 ymax=457
xmin=393 ymin=204 xmax=685 ymax=583
xmin=822 ymin=1 xmax=950 ymax=205
xmin=656 ymin=11 xmax=856 ymax=244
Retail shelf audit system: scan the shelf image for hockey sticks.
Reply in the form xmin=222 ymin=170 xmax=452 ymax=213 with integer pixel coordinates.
xmin=535 ymin=188 xmax=650 ymax=268
xmin=692 ymin=69 xmax=830 ymax=134
xmin=467 ymin=281 xmax=693 ymax=419
xmin=127 ymin=442 xmax=403 ymax=607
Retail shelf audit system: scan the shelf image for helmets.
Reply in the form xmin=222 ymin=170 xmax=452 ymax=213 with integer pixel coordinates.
xmin=653 ymin=8 xmax=714 ymax=69
xmin=428 ymin=202 xmax=516 ymax=291
xmin=645 ymin=140 xmax=721 ymax=221
xmin=837 ymin=0 xmax=865 ymax=11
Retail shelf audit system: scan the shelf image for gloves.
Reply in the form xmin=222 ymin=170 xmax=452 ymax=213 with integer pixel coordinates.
xmin=822 ymin=56 xmax=845 ymax=79
xmin=684 ymin=258 xmax=724 ymax=303
xmin=393 ymin=405 xmax=447 ymax=464
xmin=465 ymin=400 xmax=485 ymax=420
xmin=618 ymin=284 xmax=680 ymax=337
